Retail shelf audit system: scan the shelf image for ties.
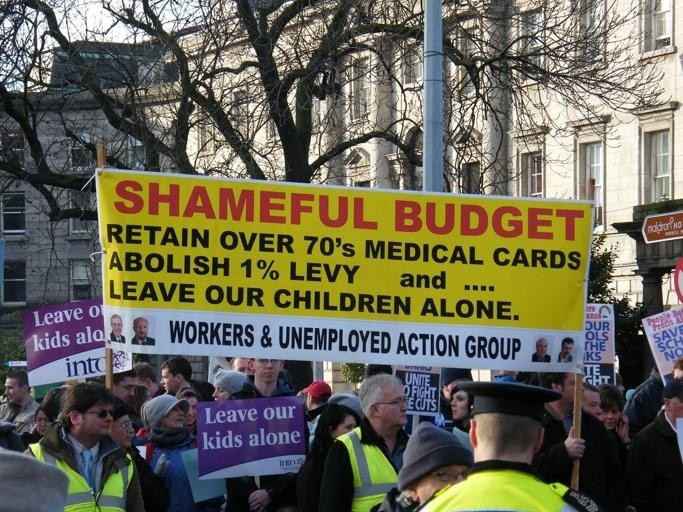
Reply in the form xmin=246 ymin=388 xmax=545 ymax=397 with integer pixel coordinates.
xmin=80 ymin=448 xmax=92 ymax=487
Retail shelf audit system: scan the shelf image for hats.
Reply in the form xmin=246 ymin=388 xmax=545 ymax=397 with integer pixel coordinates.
xmin=626 ymin=389 xmax=637 ymax=400
xmin=146 ymin=394 xmax=189 ymax=425
xmin=303 ymin=381 xmax=331 ymax=401
xmin=214 ymin=369 xmax=244 ymax=395
xmin=328 ymin=394 xmax=363 ymax=417
xmin=112 ymin=395 xmax=134 ymax=421
xmin=457 ymin=381 xmax=561 ymax=422
xmin=441 ymin=368 xmax=472 ymax=385
xmin=398 ymin=421 xmax=473 ymax=492
xmin=664 ymin=381 xmax=683 ymax=399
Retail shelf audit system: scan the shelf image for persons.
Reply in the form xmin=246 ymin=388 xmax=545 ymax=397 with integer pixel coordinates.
xmin=318 ymin=357 xmax=682 ymax=512
xmin=531 ymin=339 xmax=551 ymax=363
xmin=1 ymin=356 xmax=361 ymax=512
xmin=131 ymin=317 xmax=155 ymax=345
xmin=110 ymin=314 xmax=125 ymax=343
xmin=557 ymin=337 xmax=575 ymax=363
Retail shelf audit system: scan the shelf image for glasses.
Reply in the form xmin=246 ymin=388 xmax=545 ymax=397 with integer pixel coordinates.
xmin=87 ymin=409 xmax=115 ymax=418
xmin=123 ymin=421 xmax=132 ymax=429
xmin=375 ymin=397 xmax=408 ymax=406
xmin=431 ymin=471 xmax=466 ymax=481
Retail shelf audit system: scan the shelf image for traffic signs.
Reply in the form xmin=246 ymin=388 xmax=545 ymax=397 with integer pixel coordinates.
xmin=641 ymin=209 xmax=683 ymax=244
